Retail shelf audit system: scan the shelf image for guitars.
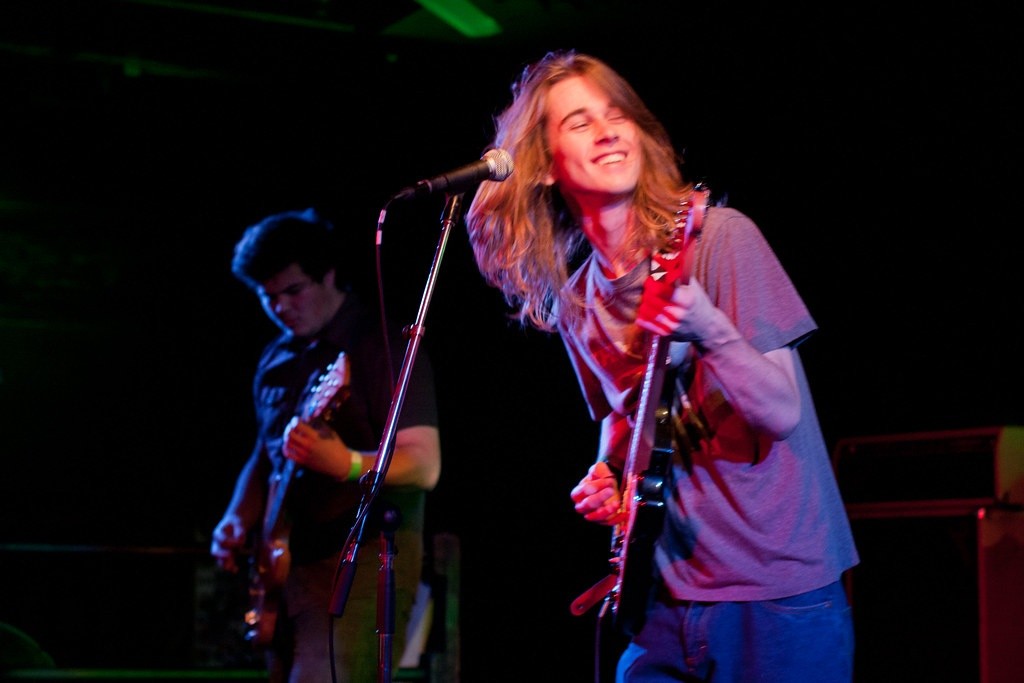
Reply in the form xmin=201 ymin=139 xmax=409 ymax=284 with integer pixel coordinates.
xmin=240 ymin=348 xmax=354 ymax=647
xmin=605 ymin=179 xmax=713 ymax=630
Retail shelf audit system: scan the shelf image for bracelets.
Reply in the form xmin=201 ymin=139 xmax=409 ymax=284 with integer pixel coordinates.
xmin=345 ymin=449 xmax=362 ymax=481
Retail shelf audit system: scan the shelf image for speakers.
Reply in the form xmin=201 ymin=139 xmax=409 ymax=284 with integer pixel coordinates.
xmin=841 ymin=509 xmax=1024 ymax=683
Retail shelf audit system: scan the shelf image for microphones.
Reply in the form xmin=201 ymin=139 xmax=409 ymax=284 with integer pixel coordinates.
xmin=415 ymin=148 xmax=515 ymax=197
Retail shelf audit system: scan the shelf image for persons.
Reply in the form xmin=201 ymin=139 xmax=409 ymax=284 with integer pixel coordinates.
xmin=464 ymin=56 xmax=858 ymax=683
xmin=207 ymin=210 xmax=442 ymax=683
